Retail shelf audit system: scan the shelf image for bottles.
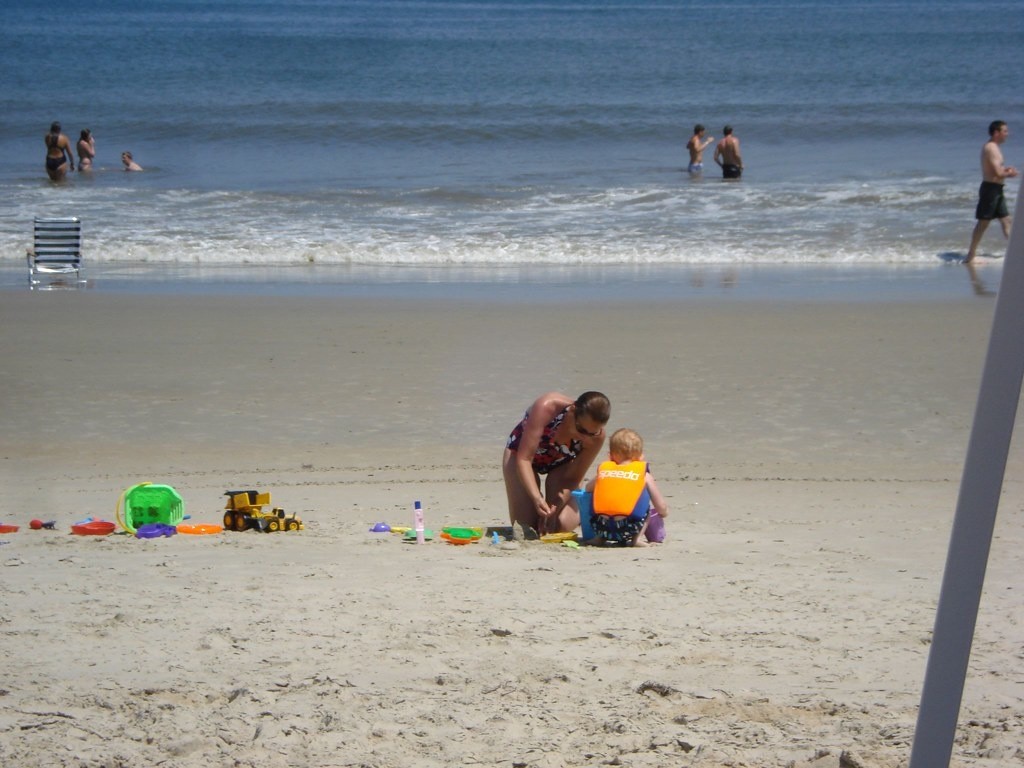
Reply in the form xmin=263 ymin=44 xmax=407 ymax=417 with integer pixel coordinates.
xmin=414 ymin=500 xmax=424 ymax=544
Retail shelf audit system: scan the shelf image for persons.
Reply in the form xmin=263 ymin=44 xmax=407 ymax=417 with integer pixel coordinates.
xmin=582 ymin=428 xmax=668 ymax=547
xmin=962 ymin=121 xmax=1016 ymax=264
xmin=77 ymin=128 xmax=94 ymax=171
xmin=714 ymin=125 xmax=744 ymax=179
xmin=502 ymin=391 xmax=611 ymax=537
xmin=45 ymin=121 xmax=75 ymax=180
xmin=122 ymin=152 xmax=143 ymax=170
xmin=687 ymin=124 xmax=714 ymax=172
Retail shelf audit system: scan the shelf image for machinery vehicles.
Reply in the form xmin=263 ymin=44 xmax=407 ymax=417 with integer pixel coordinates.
xmin=223 ymin=490 xmax=306 ymax=533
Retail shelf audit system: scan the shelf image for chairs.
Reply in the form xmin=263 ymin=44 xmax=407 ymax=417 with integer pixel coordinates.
xmin=26 ymin=216 xmax=86 ymax=285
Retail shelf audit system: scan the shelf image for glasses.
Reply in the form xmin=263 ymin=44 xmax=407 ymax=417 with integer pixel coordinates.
xmin=574 ymin=406 xmax=602 ymax=436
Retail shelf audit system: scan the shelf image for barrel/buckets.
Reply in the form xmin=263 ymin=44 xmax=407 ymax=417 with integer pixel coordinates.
xmin=116 ymin=482 xmax=184 ymax=536
